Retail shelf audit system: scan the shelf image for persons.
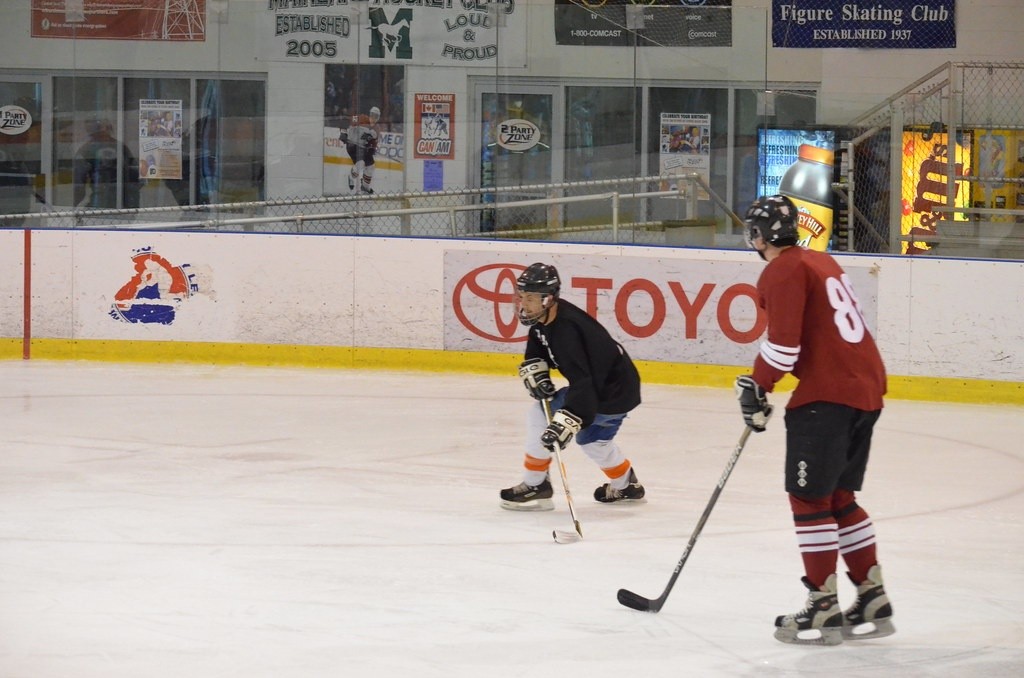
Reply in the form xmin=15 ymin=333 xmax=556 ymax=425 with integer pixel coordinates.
xmin=496 ymin=261 xmax=648 ymax=504
xmin=738 ymin=194 xmax=892 ymax=630
xmin=337 ymin=105 xmax=385 ymax=195
xmin=3 ymin=99 xmax=185 ymax=221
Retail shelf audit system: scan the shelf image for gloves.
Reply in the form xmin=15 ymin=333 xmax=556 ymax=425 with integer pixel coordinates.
xmin=737 ymin=375 xmax=772 ymax=432
xmin=518 ymin=357 xmax=554 ymax=400
xmin=540 ymin=408 xmax=584 ymax=452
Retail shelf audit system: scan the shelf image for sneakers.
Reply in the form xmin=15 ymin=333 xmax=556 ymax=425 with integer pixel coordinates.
xmin=774 ymin=572 xmax=843 ymax=630
xmin=347 ymin=173 xmax=355 ymax=190
xmin=501 ymin=474 xmax=553 ymax=503
xmin=594 ymin=467 xmax=645 ymax=503
xmin=842 ymin=566 xmax=893 ymax=627
xmin=360 ymin=182 xmax=371 ymax=192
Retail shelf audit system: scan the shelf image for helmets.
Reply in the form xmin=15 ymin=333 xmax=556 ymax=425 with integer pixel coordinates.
xmin=743 ymin=192 xmax=799 ymax=252
xmin=515 ymin=262 xmax=560 ymax=326
xmin=369 ymin=106 xmax=380 ymax=117
xmin=83 ymin=111 xmax=111 ymax=136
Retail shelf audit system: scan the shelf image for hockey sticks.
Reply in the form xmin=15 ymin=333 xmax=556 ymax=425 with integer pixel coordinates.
xmin=537 ymin=397 xmax=586 ymax=546
xmin=616 ymin=403 xmax=775 ymax=612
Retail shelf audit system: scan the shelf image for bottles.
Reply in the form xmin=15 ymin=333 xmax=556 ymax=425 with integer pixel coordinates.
xmin=780 ymin=143 xmax=835 ymax=252
